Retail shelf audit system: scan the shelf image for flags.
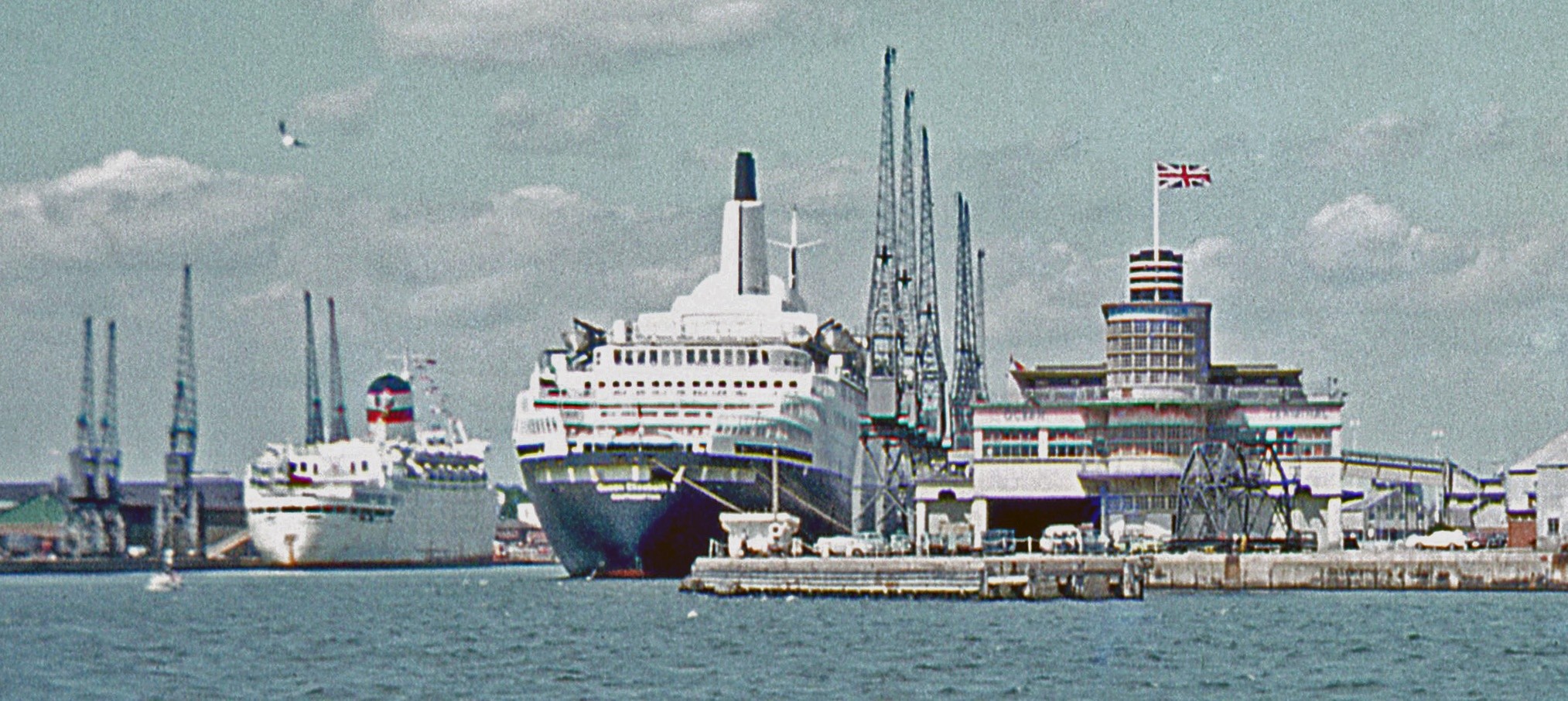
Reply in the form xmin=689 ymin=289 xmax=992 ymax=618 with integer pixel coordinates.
xmin=1156 ymin=162 xmax=1212 ymax=189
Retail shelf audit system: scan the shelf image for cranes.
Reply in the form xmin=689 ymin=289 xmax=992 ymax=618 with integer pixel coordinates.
xmin=67 ymin=315 xmax=128 ymax=558
xmin=145 ymin=262 xmax=206 ymax=559
xmin=302 ymin=288 xmax=324 ymax=444
xmin=327 ymin=299 xmax=350 ymax=442
xmin=865 ymin=44 xmax=991 ymax=449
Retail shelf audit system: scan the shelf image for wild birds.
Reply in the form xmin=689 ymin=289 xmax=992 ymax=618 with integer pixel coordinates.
xmin=278 ymin=119 xmax=308 ymax=150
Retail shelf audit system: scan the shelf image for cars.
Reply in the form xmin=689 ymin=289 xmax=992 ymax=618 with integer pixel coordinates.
xmin=813 ymin=529 xmax=975 ymax=558
xmin=1405 ymin=529 xmax=1509 ymax=549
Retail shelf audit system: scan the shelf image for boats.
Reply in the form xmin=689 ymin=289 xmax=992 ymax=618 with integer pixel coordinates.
xmin=245 ymin=345 xmax=506 ymax=565
xmin=509 ymin=150 xmax=913 ymax=579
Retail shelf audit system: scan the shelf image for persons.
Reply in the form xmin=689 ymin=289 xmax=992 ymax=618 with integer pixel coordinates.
xmin=740 ymin=528 xmax=748 ymax=558
xmin=1230 ymin=530 xmax=1248 ymax=553
